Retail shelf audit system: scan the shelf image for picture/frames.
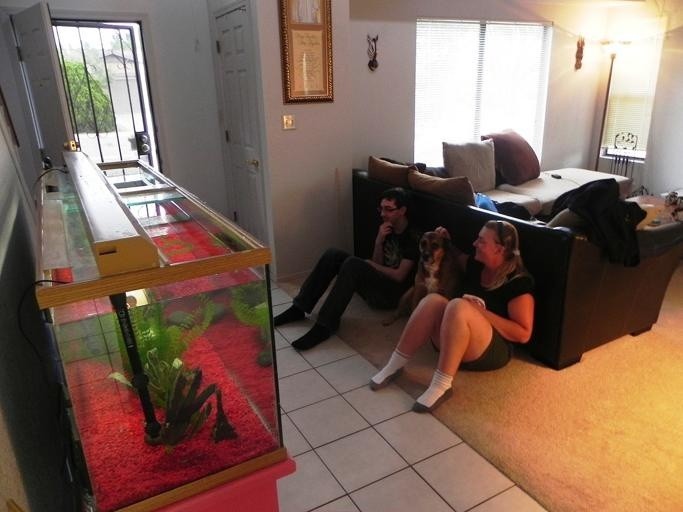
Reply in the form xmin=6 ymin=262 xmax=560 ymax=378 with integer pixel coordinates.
xmin=277 ymin=0 xmax=335 ymax=107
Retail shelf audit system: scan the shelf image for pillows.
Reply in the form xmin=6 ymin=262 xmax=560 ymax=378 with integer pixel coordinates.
xmin=480 ymin=129 xmax=540 ymax=186
xmin=441 ymin=139 xmax=495 ymax=193
xmin=407 ymin=168 xmax=475 ymax=207
xmin=368 ymin=155 xmax=418 ymax=188
xmin=381 ymin=157 xmax=426 ymax=174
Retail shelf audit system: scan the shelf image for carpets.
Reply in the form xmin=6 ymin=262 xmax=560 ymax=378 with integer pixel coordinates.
xmin=279 ymin=266 xmax=683 ymax=512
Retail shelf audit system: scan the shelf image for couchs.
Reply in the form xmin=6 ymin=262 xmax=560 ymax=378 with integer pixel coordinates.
xmin=348 ymin=161 xmax=683 ymax=370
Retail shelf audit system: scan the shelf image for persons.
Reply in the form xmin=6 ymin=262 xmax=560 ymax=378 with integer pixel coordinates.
xmin=369 ymin=219 xmax=535 ymax=412
xmin=273 ymin=188 xmax=417 ymax=350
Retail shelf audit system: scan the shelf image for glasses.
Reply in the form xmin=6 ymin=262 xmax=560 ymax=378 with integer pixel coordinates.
xmin=377 ymin=205 xmax=403 ymax=213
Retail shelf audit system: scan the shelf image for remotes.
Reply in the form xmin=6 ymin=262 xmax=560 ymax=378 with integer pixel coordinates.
xmin=551 ymin=174 xmax=561 ymax=179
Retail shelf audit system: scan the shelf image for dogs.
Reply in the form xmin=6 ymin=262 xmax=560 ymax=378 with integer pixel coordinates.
xmin=379 ymin=231 xmax=470 ymax=327
